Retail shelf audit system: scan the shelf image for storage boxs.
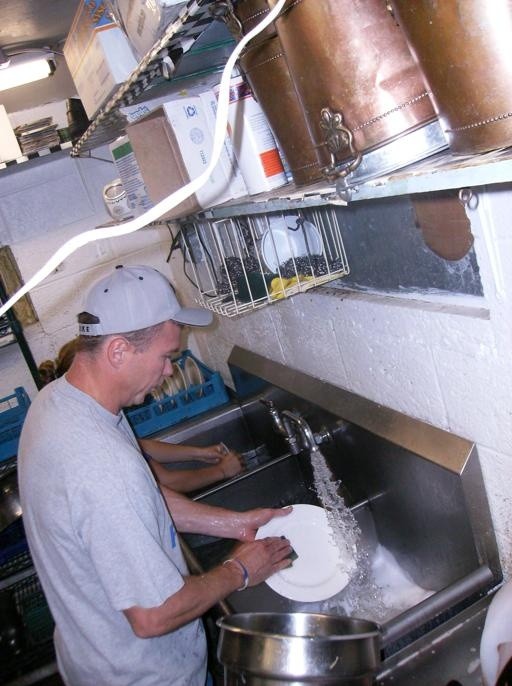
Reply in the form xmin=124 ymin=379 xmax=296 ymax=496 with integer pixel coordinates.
xmin=59 ymin=2 xmax=296 ymax=222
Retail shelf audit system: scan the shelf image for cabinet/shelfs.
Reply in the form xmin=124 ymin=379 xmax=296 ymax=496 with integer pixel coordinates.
xmin=61 ymin=1 xmax=510 ymax=229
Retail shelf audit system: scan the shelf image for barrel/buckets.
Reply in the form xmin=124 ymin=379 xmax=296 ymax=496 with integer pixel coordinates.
xmin=211 ymin=0 xmax=511 ymax=201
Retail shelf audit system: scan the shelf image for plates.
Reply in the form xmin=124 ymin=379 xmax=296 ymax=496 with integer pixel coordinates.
xmin=150 ymin=355 xmax=205 ymax=413
xmin=252 ymin=502 xmax=361 ymax=604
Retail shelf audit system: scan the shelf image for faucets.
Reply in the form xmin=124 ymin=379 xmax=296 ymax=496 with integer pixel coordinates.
xmin=258 ymin=395 xmax=284 ymax=436
xmin=281 ymin=407 xmax=320 ymax=455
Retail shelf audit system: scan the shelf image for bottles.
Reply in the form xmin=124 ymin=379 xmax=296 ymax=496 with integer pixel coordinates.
xmin=226 ymin=93 xmax=294 ymax=197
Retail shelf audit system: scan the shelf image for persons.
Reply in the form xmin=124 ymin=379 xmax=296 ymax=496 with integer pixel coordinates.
xmin=16 ymin=264 xmax=296 ymax=686
xmin=35 ymin=334 xmax=247 ymax=495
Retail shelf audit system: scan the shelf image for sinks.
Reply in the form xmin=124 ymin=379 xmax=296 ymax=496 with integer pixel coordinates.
xmin=135 ymin=401 xmax=321 ymax=498
xmin=206 ymin=497 xmax=488 ymax=684
xmin=175 ymin=444 xmax=368 ymax=580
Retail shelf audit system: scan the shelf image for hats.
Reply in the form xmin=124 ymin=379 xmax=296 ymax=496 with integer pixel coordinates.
xmin=77 ymin=263 xmax=212 ymax=345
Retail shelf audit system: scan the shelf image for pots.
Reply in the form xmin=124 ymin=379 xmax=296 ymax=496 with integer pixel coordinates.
xmin=214 ymin=611 xmax=385 ymax=686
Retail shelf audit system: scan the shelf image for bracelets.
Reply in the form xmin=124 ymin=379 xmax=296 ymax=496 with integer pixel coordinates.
xmin=220 ymin=556 xmax=251 ymax=595
xmin=217 ymin=465 xmax=227 ymax=479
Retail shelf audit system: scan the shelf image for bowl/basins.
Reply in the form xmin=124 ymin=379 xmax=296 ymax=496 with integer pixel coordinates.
xmin=253 ymin=216 xmax=324 ymax=276
